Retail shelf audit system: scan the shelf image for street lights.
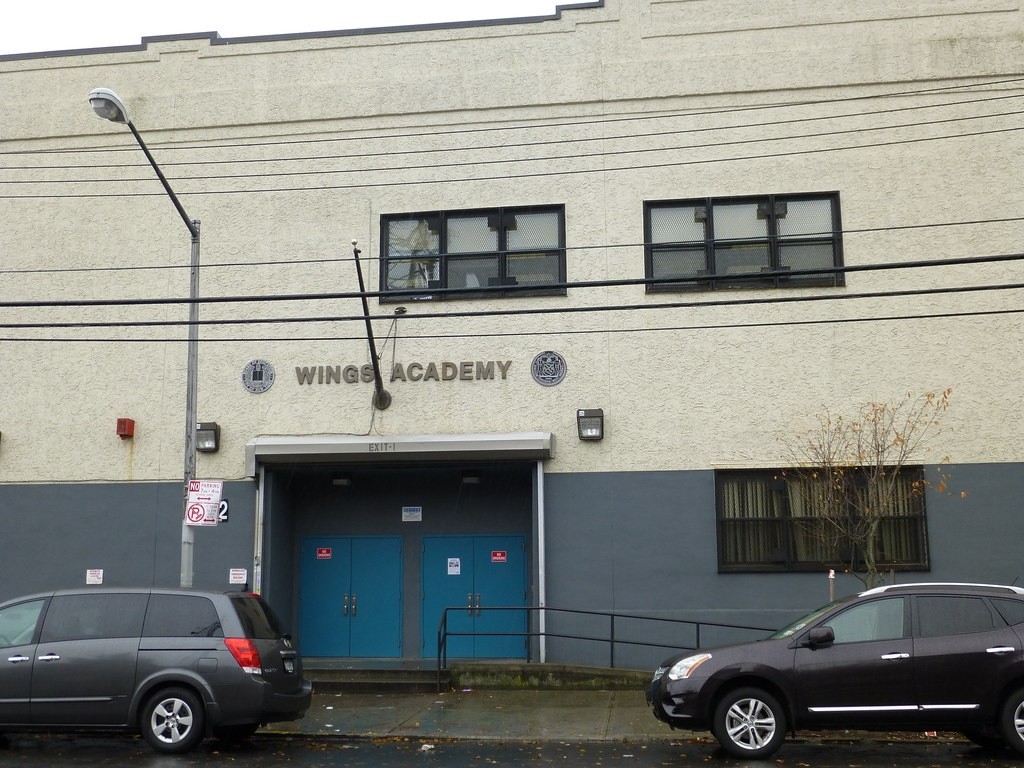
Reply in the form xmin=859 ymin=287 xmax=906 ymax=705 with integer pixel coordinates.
xmin=88 ymin=84 xmax=200 ymax=589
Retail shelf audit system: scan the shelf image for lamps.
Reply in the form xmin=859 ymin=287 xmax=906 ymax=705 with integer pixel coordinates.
xmin=196 ymin=423 xmax=220 ymax=451
xmin=576 ymin=409 xmax=604 ymax=441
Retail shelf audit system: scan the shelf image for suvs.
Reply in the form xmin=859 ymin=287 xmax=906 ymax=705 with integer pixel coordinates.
xmin=644 ymin=582 xmax=1024 ymax=756
xmin=2 ymin=587 xmax=309 ymax=748
